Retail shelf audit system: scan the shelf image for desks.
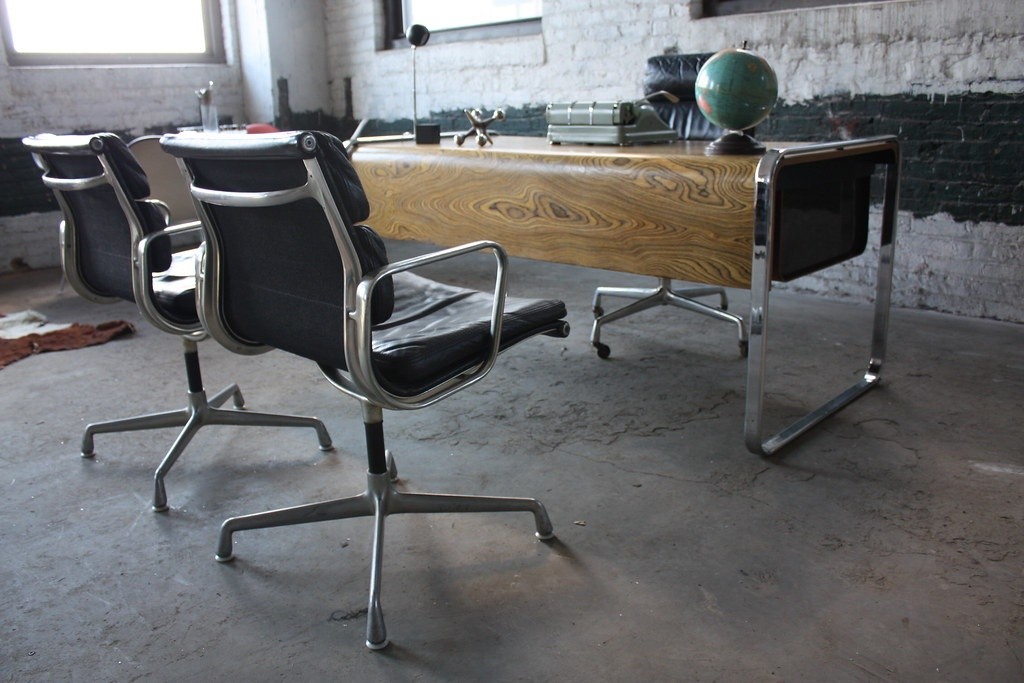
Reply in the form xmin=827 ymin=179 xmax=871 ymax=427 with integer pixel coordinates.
xmin=332 ymin=133 xmax=904 ymax=455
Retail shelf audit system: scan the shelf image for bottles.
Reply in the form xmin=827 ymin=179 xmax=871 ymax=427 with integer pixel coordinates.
xmin=199 ymin=88 xmax=218 ymax=133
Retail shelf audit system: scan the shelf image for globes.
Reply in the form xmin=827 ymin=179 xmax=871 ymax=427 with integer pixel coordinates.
xmin=695 ymin=40 xmax=778 ymax=154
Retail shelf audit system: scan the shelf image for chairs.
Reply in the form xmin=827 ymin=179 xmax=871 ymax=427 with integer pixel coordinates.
xmin=589 ymin=52 xmax=750 ymax=360
xmin=161 ymin=131 xmax=553 ymax=652
xmin=25 ymin=130 xmax=335 ymax=514
